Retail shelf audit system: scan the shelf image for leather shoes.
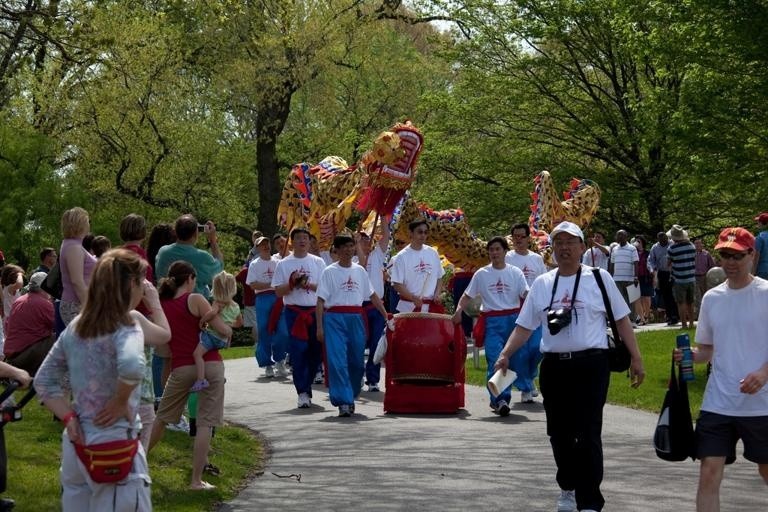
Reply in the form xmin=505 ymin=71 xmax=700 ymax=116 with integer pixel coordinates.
xmin=0 ymin=498 xmax=17 ymax=510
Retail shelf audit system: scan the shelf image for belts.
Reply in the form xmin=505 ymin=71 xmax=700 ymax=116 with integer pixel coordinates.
xmin=545 ymin=349 xmax=602 ymax=360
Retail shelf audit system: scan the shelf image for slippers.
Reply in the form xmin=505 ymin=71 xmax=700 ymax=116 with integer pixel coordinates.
xmin=189 ymin=481 xmax=217 ymax=491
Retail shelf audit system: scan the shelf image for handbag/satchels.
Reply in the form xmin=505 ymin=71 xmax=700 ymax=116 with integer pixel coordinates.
xmin=654 ymin=348 xmax=694 ymax=461
xmin=77 ymin=440 xmax=138 ymax=482
xmin=607 ymin=327 xmax=631 ymax=371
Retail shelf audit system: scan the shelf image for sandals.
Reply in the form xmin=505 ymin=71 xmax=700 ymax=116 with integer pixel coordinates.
xmin=190 ymin=379 xmax=209 ymax=391
xmin=203 ymin=464 xmax=223 ymax=476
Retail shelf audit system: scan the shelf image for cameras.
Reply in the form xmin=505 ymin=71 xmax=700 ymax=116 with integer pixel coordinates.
xmin=547 ymin=306 xmax=570 ymax=335
xmin=198 ymin=225 xmax=209 ymax=232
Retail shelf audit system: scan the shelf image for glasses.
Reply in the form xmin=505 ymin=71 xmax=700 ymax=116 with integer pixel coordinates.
xmin=719 ymin=252 xmax=750 ymax=260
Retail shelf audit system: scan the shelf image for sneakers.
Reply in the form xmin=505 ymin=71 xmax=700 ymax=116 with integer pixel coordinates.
xmin=165 ymin=414 xmax=190 ymax=432
xmin=490 ymin=387 xmax=538 ymax=416
xmin=558 ymin=489 xmax=576 ymax=511
xmin=266 ymin=354 xmax=379 ymax=416
xmin=632 ymin=312 xmax=696 ymax=330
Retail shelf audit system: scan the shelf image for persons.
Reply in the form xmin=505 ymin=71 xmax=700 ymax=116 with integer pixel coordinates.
xmin=127 ymin=277 xmax=172 ymax=462
xmin=450 ymin=224 xmax=653 ymax=416
xmin=646 ymin=225 xmax=714 ymax=329
xmin=184 ymin=389 xmax=222 ymax=477
xmin=3 ymin=208 xmax=224 ymax=374
xmin=674 ymin=227 xmax=766 ymax=512
xmin=491 ymin=218 xmax=645 ymax=512
xmin=32 ymin=248 xmax=153 ymax=511
xmin=145 ymin=261 xmax=232 ymax=494
xmin=1 ymin=361 xmax=31 ymax=512
xmin=233 ymin=218 xmax=445 ymax=418
xmin=755 ymin=212 xmax=767 ymax=281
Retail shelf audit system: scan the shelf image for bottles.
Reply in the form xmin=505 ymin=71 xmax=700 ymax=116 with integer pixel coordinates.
xmin=676 ymin=334 xmax=695 ymax=380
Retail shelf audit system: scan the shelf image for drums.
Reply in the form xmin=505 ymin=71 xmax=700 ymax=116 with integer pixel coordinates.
xmin=385 ymin=313 xmax=468 ymax=385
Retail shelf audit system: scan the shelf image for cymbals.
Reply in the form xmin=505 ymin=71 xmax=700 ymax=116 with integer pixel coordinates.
xmin=294 ymin=270 xmax=310 ymax=290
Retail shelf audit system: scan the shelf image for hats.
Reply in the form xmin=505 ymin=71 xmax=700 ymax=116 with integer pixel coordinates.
xmin=550 ymin=221 xmax=584 ymax=241
xmin=666 ymin=224 xmax=688 ymax=240
xmin=714 ymin=227 xmax=755 ymax=251
xmin=254 ymin=237 xmax=269 ymax=245
xmin=754 ymin=213 xmax=768 ymax=223
xmin=31 ymin=272 xmax=47 ymax=287
